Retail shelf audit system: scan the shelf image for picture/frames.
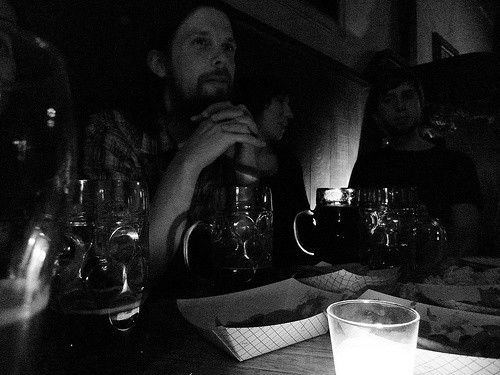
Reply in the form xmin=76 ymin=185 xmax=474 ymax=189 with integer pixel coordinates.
xmin=275 ymin=0 xmax=347 ymax=42
xmin=433 ymin=32 xmax=459 ymax=62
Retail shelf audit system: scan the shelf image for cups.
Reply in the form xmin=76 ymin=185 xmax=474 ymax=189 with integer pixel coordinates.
xmin=182 ymin=184 xmax=274 ymax=285
xmin=293 ymin=187 xmax=447 ymax=275
xmin=48 ymin=164 xmax=149 ymax=325
xmin=326 ymin=299 xmax=420 ymax=375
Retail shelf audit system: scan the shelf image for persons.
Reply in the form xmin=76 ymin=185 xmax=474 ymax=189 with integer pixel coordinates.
xmin=349 ymin=70 xmax=484 ymax=259
xmin=81 ymin=0 xmax=272 ymax=285
xmin=0 ymin=0 xmax=78 ymax=246
xmin=238 ymin=78 xmax=311 ymax=255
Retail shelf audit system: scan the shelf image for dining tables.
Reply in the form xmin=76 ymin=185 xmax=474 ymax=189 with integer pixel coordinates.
xmin=0 ymin=256 xmax=500 ymax=375
